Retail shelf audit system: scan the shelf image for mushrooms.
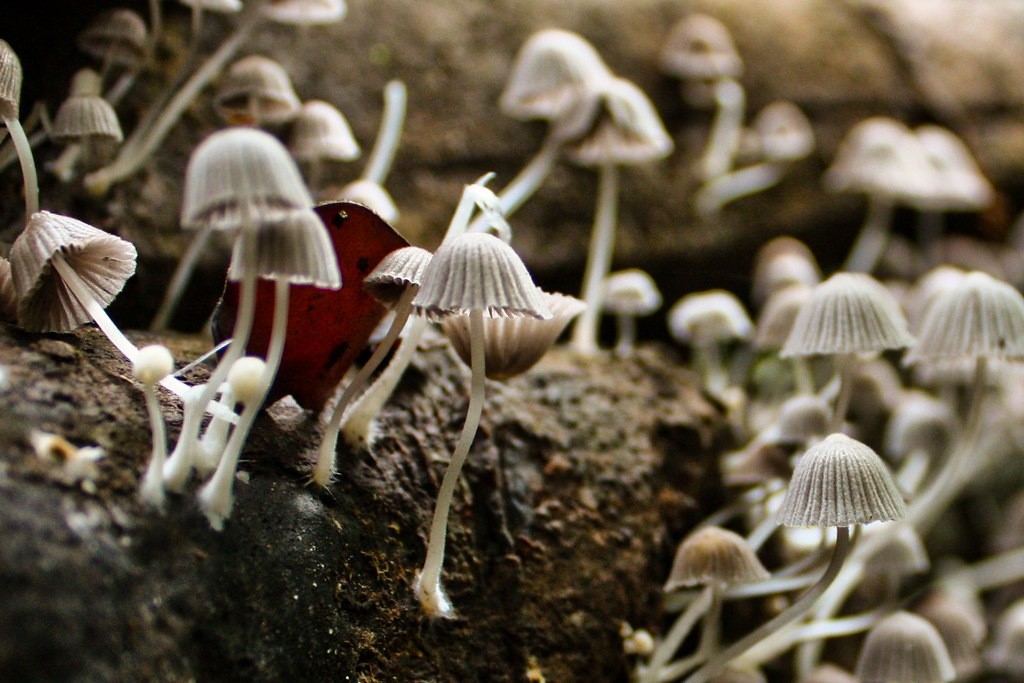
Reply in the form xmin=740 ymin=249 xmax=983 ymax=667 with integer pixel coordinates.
xmin=0 ymin=0 xmax=1024 ymax=682
